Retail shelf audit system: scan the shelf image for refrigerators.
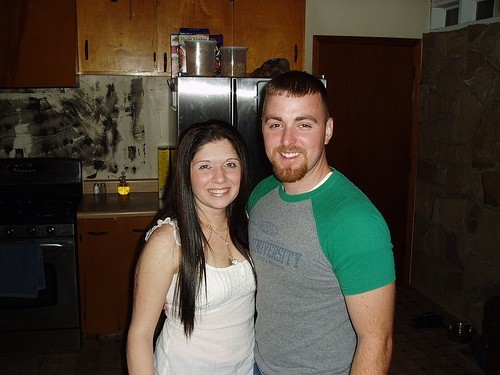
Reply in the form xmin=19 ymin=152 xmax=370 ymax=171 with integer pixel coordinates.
xmin=167 ymin=73 xmax=328 ymax=186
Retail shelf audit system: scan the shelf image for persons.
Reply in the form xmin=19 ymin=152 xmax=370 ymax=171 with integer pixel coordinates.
xmin=125 ymin=119 xmax=258 ymax=375
xmin=246 ymin=70 xmax=398 ymax=375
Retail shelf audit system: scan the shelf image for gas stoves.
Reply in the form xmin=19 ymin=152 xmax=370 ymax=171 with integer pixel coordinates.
xmin=0 ymin=157 xmax=82 ymax=239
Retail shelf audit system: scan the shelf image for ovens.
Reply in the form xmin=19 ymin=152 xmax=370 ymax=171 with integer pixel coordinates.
xmin=0 ymin=236 xmax=81 ymax=354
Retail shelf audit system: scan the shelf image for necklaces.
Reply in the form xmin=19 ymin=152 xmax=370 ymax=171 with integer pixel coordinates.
xmin=200 ymin=219 xmax=238 ymax=265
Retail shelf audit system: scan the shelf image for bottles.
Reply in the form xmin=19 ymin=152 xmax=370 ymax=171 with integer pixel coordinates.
xmin=100 ymin=183 xmax=106 ymax=195
xmin=93 ymin=183 xmax=100 ymax=195
xmin=117 ymin=171 xmax=130 ymax=195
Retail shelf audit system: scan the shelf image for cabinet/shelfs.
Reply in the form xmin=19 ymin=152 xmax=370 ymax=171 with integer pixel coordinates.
xmin=233 ymin=0 xmax=307 ymax=77
xmin=0 ymin=0 xmax=80 ymax=90
xmin=75 ymin=0 xmax=156 ymax=77
xmin=156 ymin=0 xmax=233 ymax=77
xmin=78 ymin=215 xmax=165 ymax=341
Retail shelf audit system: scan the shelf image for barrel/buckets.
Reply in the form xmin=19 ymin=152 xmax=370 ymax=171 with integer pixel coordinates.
xmin=220 ymin=47 xmax=249 ymax=77
xmin=184 ymin=40 xmax=218 ymax=77
